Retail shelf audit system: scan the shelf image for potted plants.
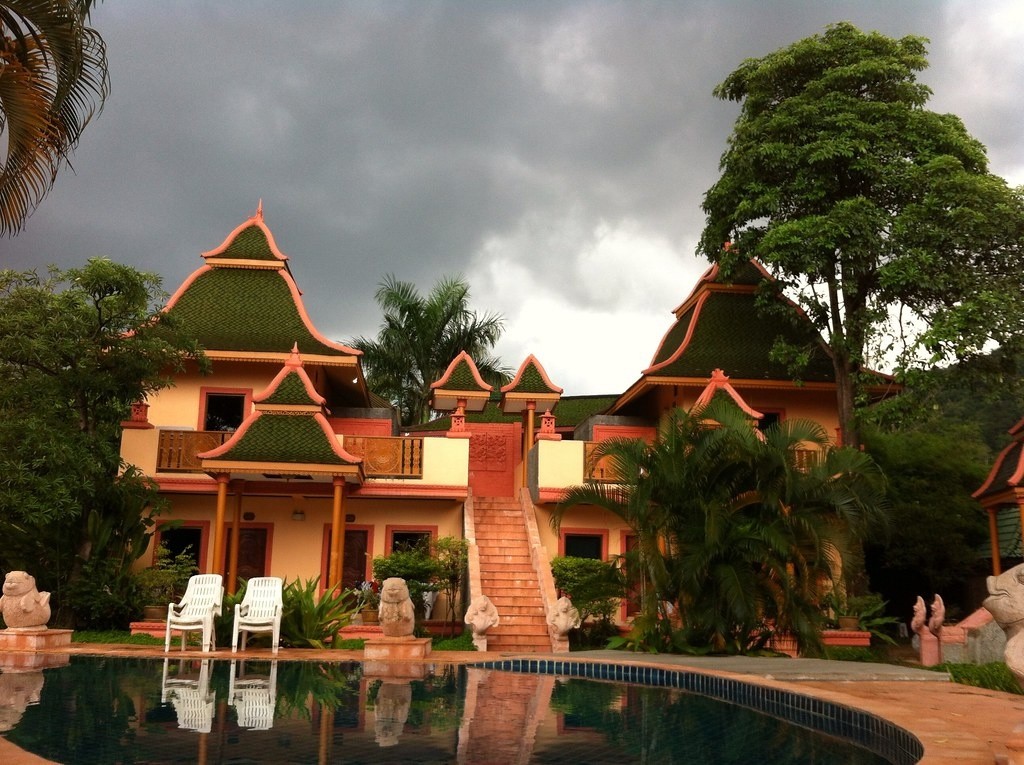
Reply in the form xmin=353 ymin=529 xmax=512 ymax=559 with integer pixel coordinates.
xmin=345 ymin=587 xmax=382 ymax=626
xmin=140 ymin=541 xmax=201 ymax=621
xmin=825 ymin=551 xmax=881 ymax=631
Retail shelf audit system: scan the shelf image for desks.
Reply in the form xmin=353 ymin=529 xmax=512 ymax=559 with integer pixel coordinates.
xmin=360 ymin=581 xmax=437 ymax=620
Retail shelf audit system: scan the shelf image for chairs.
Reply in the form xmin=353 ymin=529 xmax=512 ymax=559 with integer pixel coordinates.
xmin=231 ymin=576 xmax=284 ymax=653
xmin=164 ymin=573 xmax=225 ymax=653
xmin=161 ymin=657 xmax=216 ymax=734
xmin=228 ymin=658 xmax=277 ymax=730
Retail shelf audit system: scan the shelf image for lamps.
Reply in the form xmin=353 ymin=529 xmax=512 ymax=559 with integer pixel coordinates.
xmin=292 ymin=510 xmax=305 ymax=522
